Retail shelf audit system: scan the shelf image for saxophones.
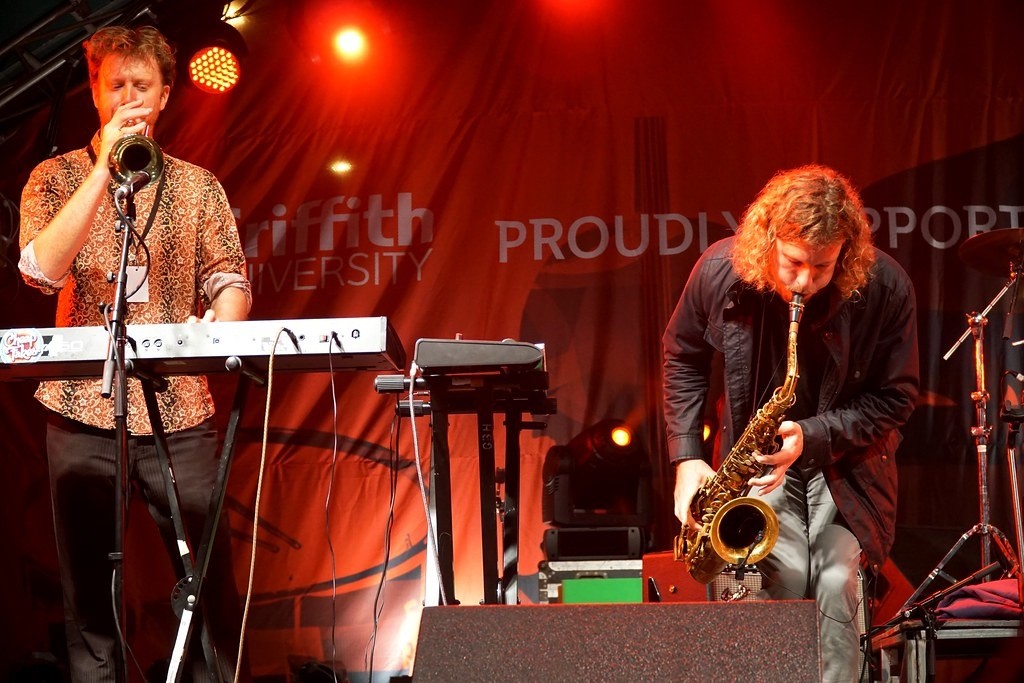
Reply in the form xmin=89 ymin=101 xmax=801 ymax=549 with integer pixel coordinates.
xmin=673 ymin=290 xmax=810 ymax=587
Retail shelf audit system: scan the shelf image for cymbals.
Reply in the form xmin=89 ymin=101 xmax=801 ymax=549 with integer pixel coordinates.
xmin=953 ymin=225 xmax=1024 ymax=291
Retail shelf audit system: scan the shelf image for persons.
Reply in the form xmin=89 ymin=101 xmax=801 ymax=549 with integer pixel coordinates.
xmin=661 ymin=164 xmax=920 ymax=683
xmin=18 ymin=27 xmax=252 ymax=683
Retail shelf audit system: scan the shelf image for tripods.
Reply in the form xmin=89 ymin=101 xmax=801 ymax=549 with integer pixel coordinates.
xmin=894 ymin=257 xmax=1023 ymax=616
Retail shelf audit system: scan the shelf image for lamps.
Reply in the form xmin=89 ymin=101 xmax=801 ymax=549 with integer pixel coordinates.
xmin=185 ymin=22 xmax=242 ymax=93
xmin=543 ymin=417 xmax=651 ymax=527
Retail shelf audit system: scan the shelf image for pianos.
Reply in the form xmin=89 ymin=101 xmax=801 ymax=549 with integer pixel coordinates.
xmin=0 ymin=307 xmax=409 ymax=683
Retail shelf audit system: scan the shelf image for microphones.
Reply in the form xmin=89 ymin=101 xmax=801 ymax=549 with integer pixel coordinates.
xmin=116 ymin=170 xmax=151 ymax=199
xmin=735 ymin=557 xmax=747 ymax=580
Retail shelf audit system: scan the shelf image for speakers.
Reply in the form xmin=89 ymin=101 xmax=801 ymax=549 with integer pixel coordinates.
xmin=411 ymin=599 xmax=823 ymax=683
xmin=643 ymin=553 xmax=763 ymax=602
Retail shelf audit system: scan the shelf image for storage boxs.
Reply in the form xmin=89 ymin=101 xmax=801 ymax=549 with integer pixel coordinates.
xmin=537 ymin=558 xmax=644 ymax=606
